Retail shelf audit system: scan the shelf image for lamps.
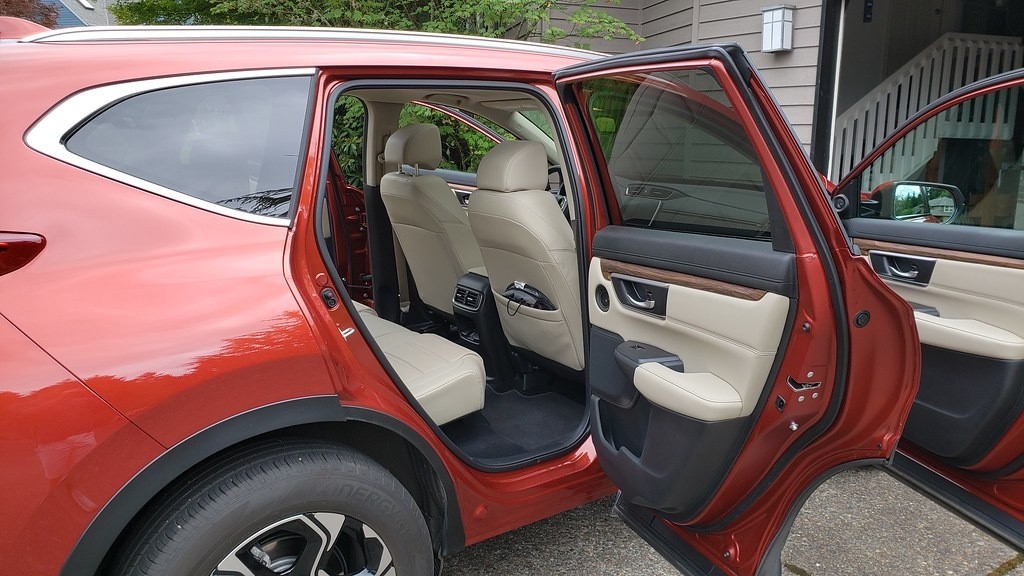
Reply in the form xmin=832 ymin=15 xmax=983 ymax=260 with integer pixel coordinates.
xmin=760 ymin=4 xmax=796 ymax=54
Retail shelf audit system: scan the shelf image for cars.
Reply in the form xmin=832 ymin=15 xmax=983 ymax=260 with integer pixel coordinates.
xmin=1 ymin=24 xmax=1024 ymax=576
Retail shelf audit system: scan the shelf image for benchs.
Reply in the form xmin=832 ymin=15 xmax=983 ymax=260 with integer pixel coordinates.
xmin=350 ymin=298 xmax=487 ymax=426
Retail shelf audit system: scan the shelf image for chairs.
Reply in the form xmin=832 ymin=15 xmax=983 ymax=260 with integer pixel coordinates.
xmin=467 ymin=140 xmax=585 ymax=371
xmin=379 ymin=123 xmax=485 ymax=315
